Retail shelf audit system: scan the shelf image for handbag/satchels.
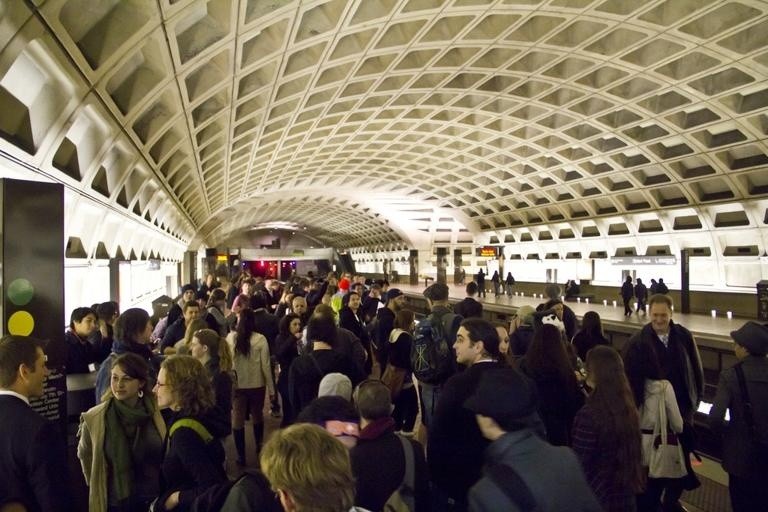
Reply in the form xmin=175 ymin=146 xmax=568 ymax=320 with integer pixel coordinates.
xmin=380 ymin=364 xmax=407 ymax=393
xmin=413 ymin=415 xmax=427 ymax=447
xmin=384 ymin=485 xmax=416 ymax=512
xmin=647 ymin=442 xmax=689 ymax=479
xmin=721 ymin=420 xmax=759 ymax=474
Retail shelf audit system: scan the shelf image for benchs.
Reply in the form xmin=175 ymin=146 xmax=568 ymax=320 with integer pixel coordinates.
xmin=570 ymin=293 xmax=595 ymax=303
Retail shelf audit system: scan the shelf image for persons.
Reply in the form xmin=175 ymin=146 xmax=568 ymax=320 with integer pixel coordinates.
xmin=476 ymin=268 xmax=514 ymax=298
xmin=708 ymin=321 xmax=768 ymax=512
xmin=62 ymin=259 xmax=387 ymax=512
xmin=620 ymin=275 xmax=668 ymax=317
xmin=368 ymin=280 xmax=703 ymax=512
xmin=0 ymin=335 xmax=65 ymax=510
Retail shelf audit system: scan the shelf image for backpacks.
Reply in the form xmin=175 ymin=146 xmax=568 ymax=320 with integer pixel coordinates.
xmin=409 ymin=310 xmax=457 ymax=386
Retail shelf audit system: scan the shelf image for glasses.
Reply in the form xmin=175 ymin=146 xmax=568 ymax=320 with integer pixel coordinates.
xmin=156 ymin=380 xmax=175 ymax=387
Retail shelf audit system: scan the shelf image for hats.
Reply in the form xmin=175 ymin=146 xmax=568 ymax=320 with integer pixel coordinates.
xmin=516 ymin=305 xmax=536 ymax=325
xmin=730 ymin=322 xmax=768 ymax=355
xmin=318 ymin=373 xmax=353 ymax=402
xmin=386 ymin=289 xmax=404 ymax=299
xmin=424 ymin=284 xmax=448 ymax=300
xmin=462 ymin=370 xmax=537 ymax=418
xmin=338 ymin=280 xmax=350 ymax=289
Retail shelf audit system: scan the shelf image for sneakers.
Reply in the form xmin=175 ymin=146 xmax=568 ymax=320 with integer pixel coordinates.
xmin=268 ymin=408 xmax=282 ymax=418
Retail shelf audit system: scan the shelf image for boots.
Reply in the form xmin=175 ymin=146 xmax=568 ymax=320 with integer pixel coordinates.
xmin=253 ymin=423 xmax=264 ymax=454
xmin=233 ymin=428 xmax=246 ymax=465
